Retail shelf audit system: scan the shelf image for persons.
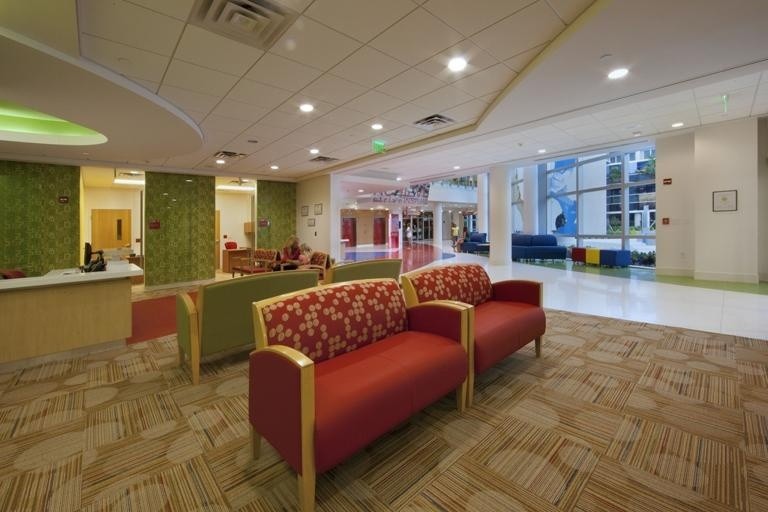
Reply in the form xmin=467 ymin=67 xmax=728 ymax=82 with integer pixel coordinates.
xmin=452 ymin=226 xmax=471 ymax=252
xmin=405 ymin=223 xmax=412 ymax=249
xmin=286 ymin=242 xmax=312 ymax=270
xmin=280 ymin=235 xmax=302 ymax=263
xmin=452 ymin=223 xmax=460 ymax=247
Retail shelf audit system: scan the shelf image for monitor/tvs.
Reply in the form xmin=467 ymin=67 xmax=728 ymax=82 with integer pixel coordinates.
xmin=84 ymin=243 xmax=92 ymax=265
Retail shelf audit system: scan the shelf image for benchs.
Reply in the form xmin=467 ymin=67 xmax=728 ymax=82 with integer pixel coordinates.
xmin=247 ymin=277 xmax=469 ymax=511
xmin=174 ymin=256 xmax=403 ymax=388
xmin=399 ymin=255 xmax=547 ymax=409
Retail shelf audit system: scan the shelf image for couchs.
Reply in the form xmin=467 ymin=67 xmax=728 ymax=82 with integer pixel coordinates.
xmin=512 ymin=233 xmax=567 ymax=263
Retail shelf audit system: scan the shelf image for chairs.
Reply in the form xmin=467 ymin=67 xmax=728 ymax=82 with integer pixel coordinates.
xmin=461 ymin=231 xmax=487 ymax=254
xmin=232 ymin=234 xmax=330 ymax=283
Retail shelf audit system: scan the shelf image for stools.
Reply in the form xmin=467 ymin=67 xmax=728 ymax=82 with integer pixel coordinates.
xmin=477 ymin=243 xmax=489 ymax=256
